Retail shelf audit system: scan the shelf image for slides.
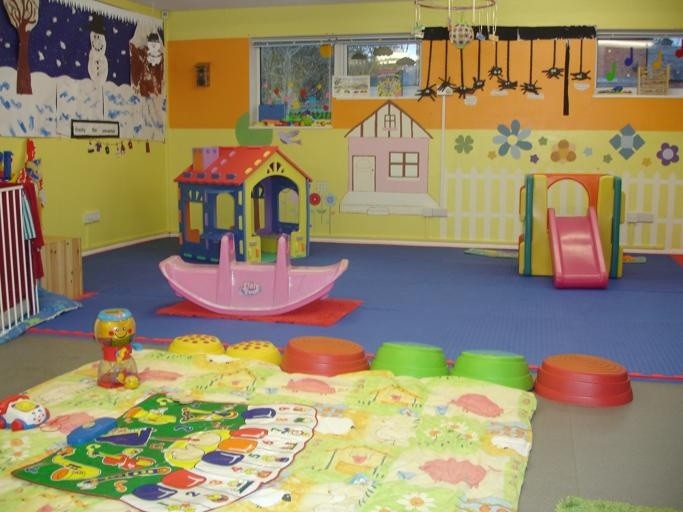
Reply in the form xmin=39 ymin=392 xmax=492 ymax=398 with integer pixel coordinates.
xmin=545 ymin=207 xmax=610 ymax=287
